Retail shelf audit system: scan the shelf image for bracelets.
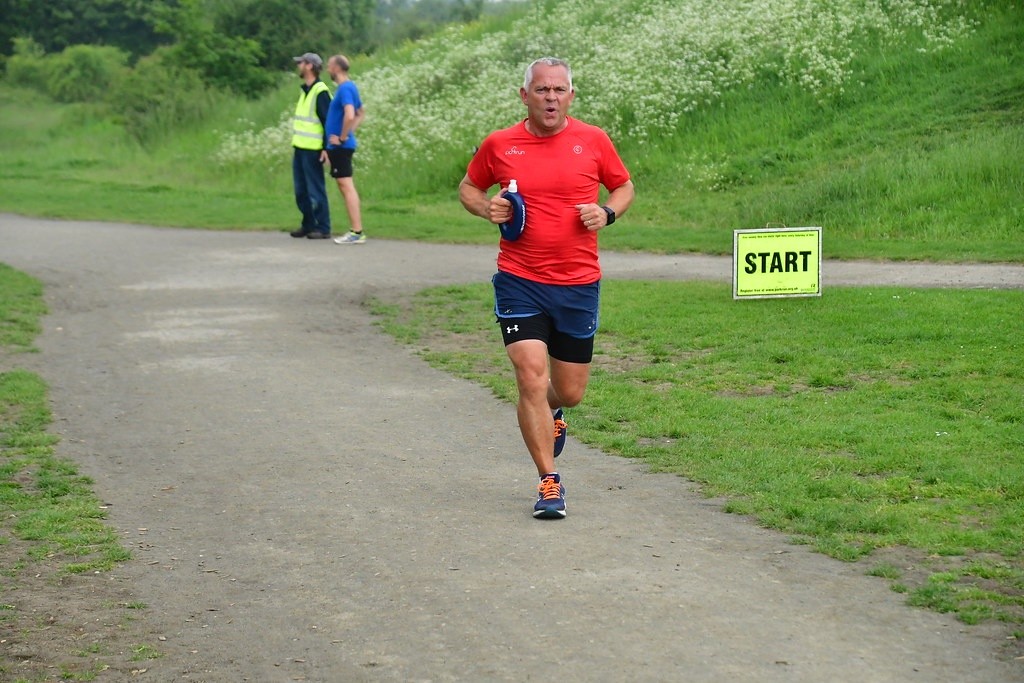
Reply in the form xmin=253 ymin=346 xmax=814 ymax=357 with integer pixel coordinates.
xmin=338 ymin=138 xmax=346 ymax=143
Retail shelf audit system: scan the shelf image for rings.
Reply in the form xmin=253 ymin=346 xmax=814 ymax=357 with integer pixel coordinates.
xmin=588 ymin=220 xmax=591 ymax=226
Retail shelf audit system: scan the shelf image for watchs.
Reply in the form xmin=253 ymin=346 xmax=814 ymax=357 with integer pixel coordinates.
xmin=601 ymin=206 xmax=615 ymax=226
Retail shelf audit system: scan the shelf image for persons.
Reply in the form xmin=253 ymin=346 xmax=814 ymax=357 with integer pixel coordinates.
xmin=458 ymin=57 xmax=635 ymax=521
xmin=324 ymin=54 xmax=367 ymax=244
xmin=288 ymin=52 xmax=333 ymax=239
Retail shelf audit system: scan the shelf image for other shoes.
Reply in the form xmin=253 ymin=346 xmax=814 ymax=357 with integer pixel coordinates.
xmin=291 ymin=228 xmax=309 ymax=237
xmin=308 ymin=230 xmax=330 ymax=238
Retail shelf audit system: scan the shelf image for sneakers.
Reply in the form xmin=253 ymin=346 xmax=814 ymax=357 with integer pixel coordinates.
xmin=532 ymin=472 xmax=566 ymax=518
xmin=334 ymin=230 xmax=366 ymax=244
xmin=553 ymin=407 xmax=567 ymax=457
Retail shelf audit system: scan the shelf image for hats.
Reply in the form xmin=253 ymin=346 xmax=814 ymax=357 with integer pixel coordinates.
xmin=293 ymin=52 xmax=323 ymax=68
